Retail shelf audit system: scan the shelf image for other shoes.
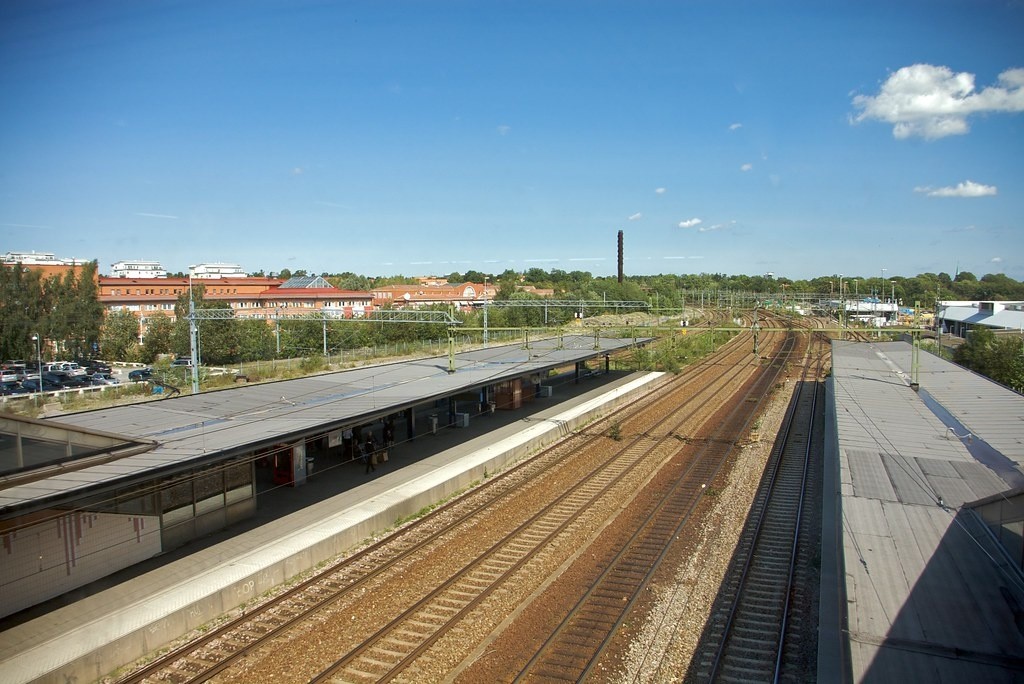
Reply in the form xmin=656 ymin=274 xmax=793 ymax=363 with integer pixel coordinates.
xmin=366 ymin=472 xmax=370 ymax=475
xmin=372 ymin=468 xmax=376 ymax=471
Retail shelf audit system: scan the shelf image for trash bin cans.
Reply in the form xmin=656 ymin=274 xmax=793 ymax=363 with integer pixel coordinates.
xmin=487 ymin=401 xmax=496 ymax=413
xmin=428 ymin=413 xmax=438 ymax=434
xmin=306 ymin=457 xmax=315 ymax=475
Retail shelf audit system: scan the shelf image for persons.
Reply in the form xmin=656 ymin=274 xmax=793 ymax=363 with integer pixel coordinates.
xmin=367 ymin=430 xmax=380 ymax=453
xmin=383 ymin=420 xmax=395 ymax=447
xmin=353 ymin=439 xmax=367 ymax=464
xmin=365 ymin=438 xmax=376 ymax=474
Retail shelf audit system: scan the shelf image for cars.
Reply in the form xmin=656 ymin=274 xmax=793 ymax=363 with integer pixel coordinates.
xmin=85 ymin=373 xmax=120 ymax=385
xmin=144 ymin=366 xmax=156 ymax=374
xmin=169 ymin=358 xmax=192 ymax=369
xmin=127 ymin=370 xmax=150 ymax=383
xmin=0 ymin=358 xmax=113 ymax=397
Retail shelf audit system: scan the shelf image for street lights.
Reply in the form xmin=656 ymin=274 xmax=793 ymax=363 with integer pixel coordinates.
xmin=881 ymin=268 xmax=887 ymax=318
xmin=189 ymin=265 xmax=199 ymax=300
xmin=483 ymin=276 xmax=489 ymax=342
xmin=31 ymin=331 xmax=44 ymax=398
xmin=839 ymin=273 xmax=843 ymax=301
xmin=891 ymin=279 xmax=897 ymax=303
xmin=853 ymin=279 xmax=858 ymax=298
xmin=830 ymin=280 xmax=834 ymax=294
